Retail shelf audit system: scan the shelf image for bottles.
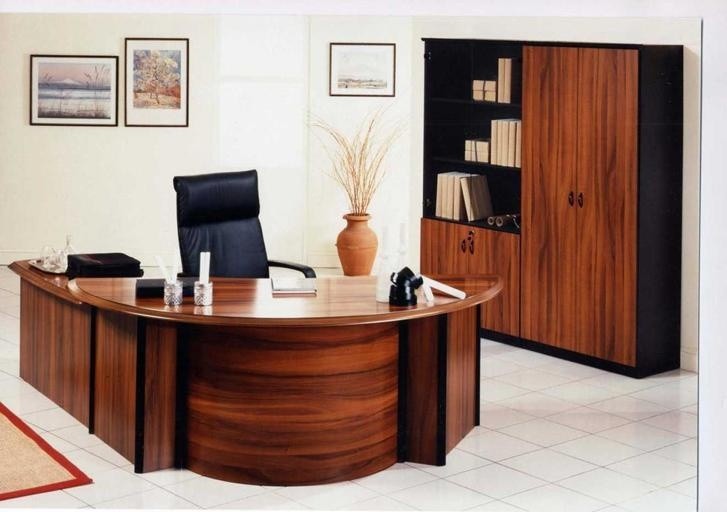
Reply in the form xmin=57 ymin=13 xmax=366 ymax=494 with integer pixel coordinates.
xmin=58 ymin=234 xmax=76 ymax=270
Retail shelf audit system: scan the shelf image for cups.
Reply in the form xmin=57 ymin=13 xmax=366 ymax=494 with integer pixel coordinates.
xmin=166 ymin=279 xmax=211 ymax=306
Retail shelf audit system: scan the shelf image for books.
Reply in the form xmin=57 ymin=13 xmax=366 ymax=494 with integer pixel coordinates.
xmin=434 ymin=56 xmax=521 ymax=222
xmin=271 ymin=274 xmax=318 ymax=295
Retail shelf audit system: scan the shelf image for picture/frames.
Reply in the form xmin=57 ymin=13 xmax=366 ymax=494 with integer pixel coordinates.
xmin=29 ymin=54 xmax=120 ymax=127
xmin=330 ymin=42 xmax=397 ymax=97
xmin=124 ymin=36 xmax=189 ymax=128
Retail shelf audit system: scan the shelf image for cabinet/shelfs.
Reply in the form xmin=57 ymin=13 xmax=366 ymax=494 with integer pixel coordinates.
xmin=420 ymin=36 xmax=681 ymax=379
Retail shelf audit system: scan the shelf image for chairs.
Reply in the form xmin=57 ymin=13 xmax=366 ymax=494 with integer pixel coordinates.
xmin=173 ymin=170 xmax=317 ymax=279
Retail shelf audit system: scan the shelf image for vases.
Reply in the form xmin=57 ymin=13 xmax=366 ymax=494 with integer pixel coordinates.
xmin=337 ymin=213 xmax=378 ymax=275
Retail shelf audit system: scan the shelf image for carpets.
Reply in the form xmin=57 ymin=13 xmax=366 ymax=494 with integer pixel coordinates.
xmin=1 ymin=402 xmax=92 ymax=501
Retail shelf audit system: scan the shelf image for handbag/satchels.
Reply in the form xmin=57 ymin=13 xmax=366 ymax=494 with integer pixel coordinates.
xmin=67 ymin=253 xmax=144 ymax=280
